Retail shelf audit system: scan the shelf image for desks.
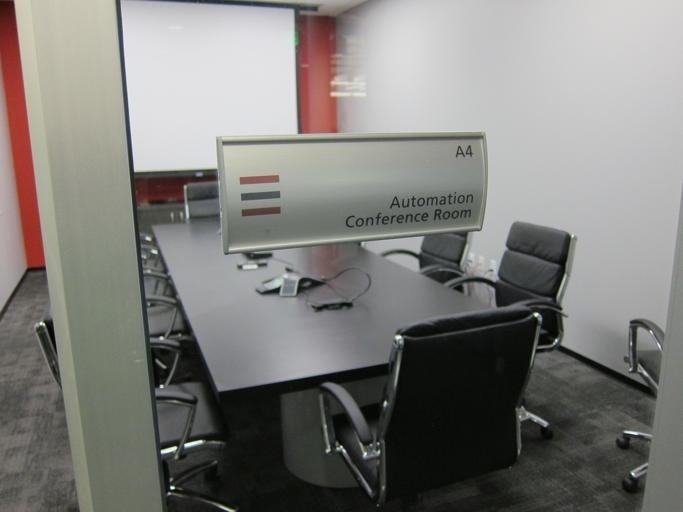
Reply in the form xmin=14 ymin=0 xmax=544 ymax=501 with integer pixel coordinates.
xmin=147 ymin=214 xmax=501 ymax=512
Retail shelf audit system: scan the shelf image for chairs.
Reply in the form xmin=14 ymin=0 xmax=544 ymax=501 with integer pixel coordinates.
xmin=375 ymin=228 xmax=476 ymax=298
xmin=184 ymin=180 xmax=222 ymax=218
xmin=314 ymin=303 xmax=545 ymax=512
xmin=31 ymin=314 xmax=243 ymax=512
xmin=131 ymin=220 xmax=202 ymax=385
xmin=609 ymin=311 xmax=667 ymax=494
xmin=440 ymin=220 xmax=579 ymax=441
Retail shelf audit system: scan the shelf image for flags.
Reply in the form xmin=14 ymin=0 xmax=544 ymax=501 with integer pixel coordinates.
xmin=239 ymin=174 xmax=282 ymax=216
